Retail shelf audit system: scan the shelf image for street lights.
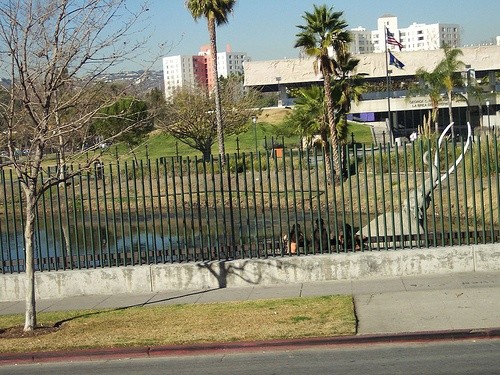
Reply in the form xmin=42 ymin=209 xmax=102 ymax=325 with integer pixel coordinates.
xmin=100 ymin=144 xmax=107 ymax=182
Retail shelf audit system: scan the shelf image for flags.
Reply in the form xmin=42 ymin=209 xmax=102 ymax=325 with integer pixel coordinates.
xmin=388 ymin=50 xmax=404 ymax=70
xmin=386 ymin=29 xmax=403 ymax=50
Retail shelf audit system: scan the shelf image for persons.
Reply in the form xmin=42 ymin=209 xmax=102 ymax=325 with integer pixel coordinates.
xmin=96 ymin=159 xmax=104 ymax=177
xmin=283 ymin=223 xmax=308 ymax=254
xmin=310 ymin=218 xmax=331 ymax=253
xmin=333 ymin=223 xmax=359 ymax=250
